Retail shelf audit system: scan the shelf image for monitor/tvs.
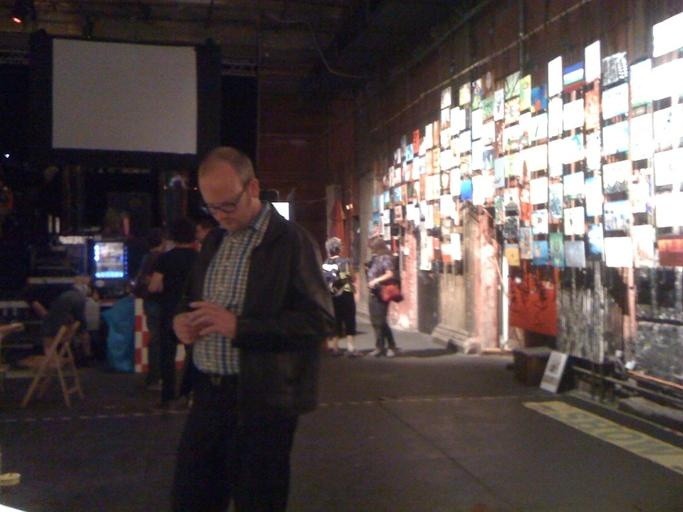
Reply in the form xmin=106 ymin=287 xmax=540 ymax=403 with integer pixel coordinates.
xmin=88 ymin=239 xmax=128 ymax=280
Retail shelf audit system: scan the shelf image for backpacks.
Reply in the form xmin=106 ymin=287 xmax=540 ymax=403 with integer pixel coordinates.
xmin=320 ymin=256 xmax=350 ymax=297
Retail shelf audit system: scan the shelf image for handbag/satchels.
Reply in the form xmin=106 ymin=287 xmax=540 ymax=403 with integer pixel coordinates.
xmin=380 ymin=284 xmax=404 ymax=303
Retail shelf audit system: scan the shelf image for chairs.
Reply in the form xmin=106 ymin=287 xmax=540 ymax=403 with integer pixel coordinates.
xmin=17 ymin=322 xmax=84 ymax=410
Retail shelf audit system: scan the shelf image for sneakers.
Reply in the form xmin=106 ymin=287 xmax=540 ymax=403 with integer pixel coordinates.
xmin=365 ymin=347 xmax=394 ymax=358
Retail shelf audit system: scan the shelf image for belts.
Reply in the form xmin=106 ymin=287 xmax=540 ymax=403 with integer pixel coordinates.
xmin=203 ymin=372 xmax=240 ymax=388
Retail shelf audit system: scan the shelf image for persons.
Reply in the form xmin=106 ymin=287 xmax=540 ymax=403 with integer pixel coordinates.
xmin=320 ymin=236 xmax=363 ymax=357
xmin=172 ymin=146 xmax=334 ymax=512
xmin=367 ymin=236 xmax=400 ymax=357
xmin=24 ymin=212 xmax=226 ymax=409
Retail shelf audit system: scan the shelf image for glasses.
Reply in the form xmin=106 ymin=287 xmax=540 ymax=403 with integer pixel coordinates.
xmin=201 ymin=181 xmax=250 ymax=215
